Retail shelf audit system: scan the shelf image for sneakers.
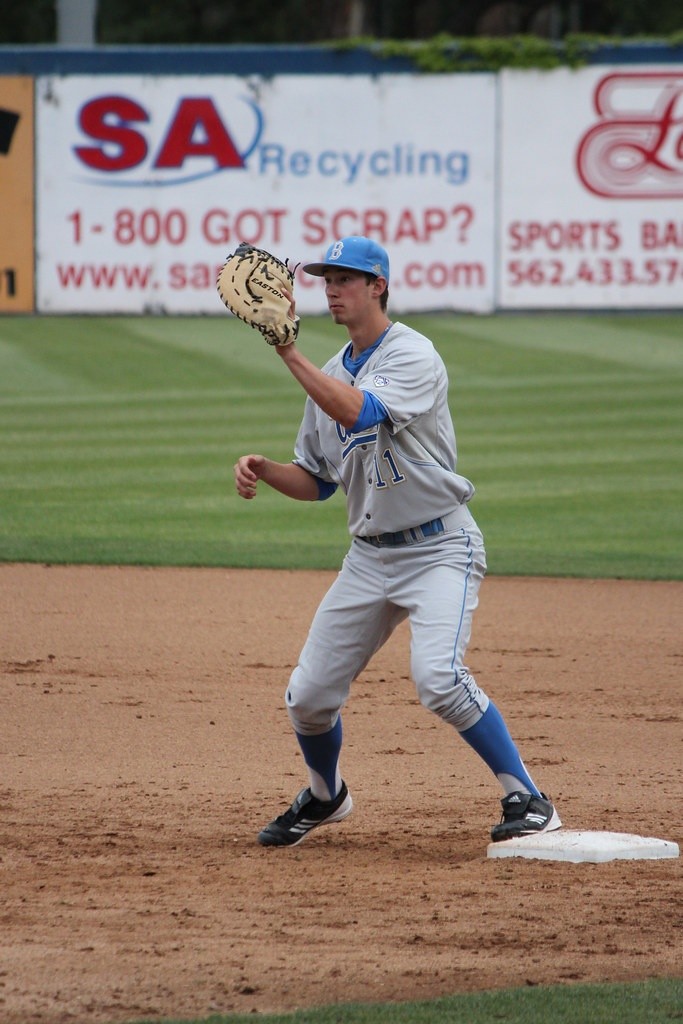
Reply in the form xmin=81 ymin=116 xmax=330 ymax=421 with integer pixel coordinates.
xmin=257 ymin=779 xmax=353 ymax=848
xmin=491 ymin=790 xmax=562 ymax=843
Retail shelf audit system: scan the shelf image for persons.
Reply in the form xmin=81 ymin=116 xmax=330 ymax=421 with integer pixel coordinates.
xmin=234 ymin=236 xmax=564 ymax=852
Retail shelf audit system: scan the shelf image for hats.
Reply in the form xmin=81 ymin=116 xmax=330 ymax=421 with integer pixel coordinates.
xmin=302 ymin=236 xmax=390 ymax=287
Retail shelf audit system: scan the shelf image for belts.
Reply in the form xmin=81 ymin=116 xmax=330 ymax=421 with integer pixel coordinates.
xmin=356 ymin=517 xmax=444 ymax=548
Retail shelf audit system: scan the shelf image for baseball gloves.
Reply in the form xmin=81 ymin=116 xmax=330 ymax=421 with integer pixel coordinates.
xmin=216 ymin=240 xmax=301 ymax=346
